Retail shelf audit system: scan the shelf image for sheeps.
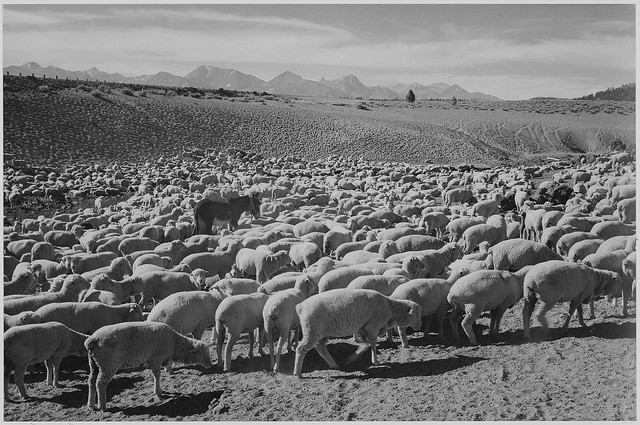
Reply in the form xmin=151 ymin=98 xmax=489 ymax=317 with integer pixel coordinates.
xmin=3 ymin=146 xmax=636 ymax=412
